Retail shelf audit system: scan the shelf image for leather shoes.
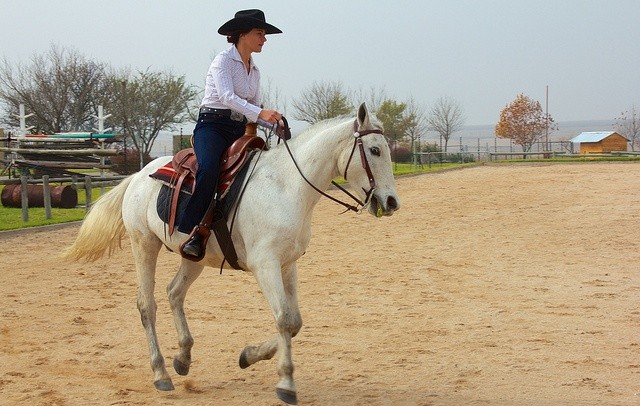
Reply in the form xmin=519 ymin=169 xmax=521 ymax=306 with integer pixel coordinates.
xmin=183 ymin=236 xmax=202 ymax=256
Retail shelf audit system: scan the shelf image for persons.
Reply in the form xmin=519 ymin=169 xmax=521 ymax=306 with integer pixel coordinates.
xmin=183 ymin=10 xmax=286 ymax=257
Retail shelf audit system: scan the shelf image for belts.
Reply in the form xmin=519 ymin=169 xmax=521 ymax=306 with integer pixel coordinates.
xmin=199 ymin=108 xmax=247 ymax=121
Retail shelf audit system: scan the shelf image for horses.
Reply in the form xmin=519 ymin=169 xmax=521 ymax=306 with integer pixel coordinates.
xmin=65 ymin=101 xmax=399 ymax=406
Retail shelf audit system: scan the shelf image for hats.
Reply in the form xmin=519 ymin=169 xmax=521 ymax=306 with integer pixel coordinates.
xmin=218 ymin=9 xmax=282 ymax=34
